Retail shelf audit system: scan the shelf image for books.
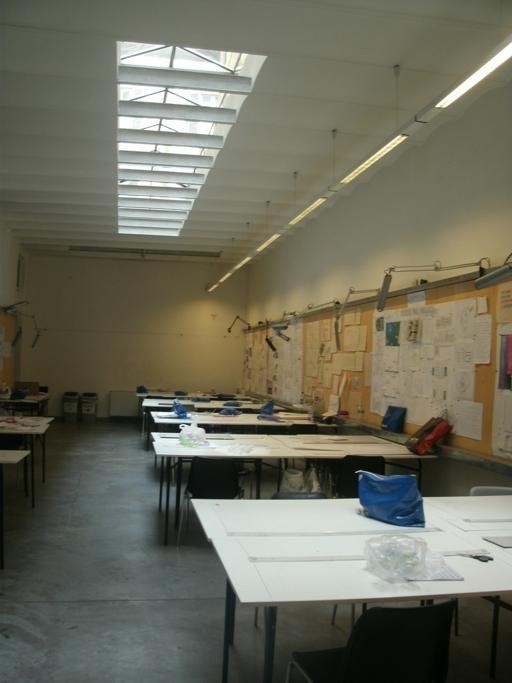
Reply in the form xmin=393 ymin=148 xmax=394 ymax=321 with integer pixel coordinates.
xmin=279 ymin=411 xmax=311 ymax=420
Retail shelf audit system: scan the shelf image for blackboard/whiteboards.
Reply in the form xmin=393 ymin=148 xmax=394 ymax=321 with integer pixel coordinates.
xmin=243 ymin=299 xmax=361 ymax=424
xmin=359 ymin=263 xmax=512 ymax=475
xmin=109 ymin=391 xmax=139 ymax=417
xmin=236 ymin=262 xmax=512 ymax=476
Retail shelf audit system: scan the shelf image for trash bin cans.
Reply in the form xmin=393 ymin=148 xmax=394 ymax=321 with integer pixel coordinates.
xmin=80 ymin=392 xmax=98 ymax=422
xmin=63 ymin=391 xmax=80 ymax=422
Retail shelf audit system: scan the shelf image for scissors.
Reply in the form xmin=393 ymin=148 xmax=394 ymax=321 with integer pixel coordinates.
xmin=458 ymin=554 xmax=493 ymax=562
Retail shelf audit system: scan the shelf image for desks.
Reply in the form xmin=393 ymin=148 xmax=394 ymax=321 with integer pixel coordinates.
xmin=136 ymin=383 xmax=358 ymax=449
xmin=1 ymin=385 xmax=52 ymax=508
xmin=149 ymin=435 xmax=438 ymax=546
xmin=190 ymin=495 xmax=512 ymax=683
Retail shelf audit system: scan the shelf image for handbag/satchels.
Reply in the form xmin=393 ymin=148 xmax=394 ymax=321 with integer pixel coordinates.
xmin=381 ymin=406 xmax=406 ymax=432
xmin=355 ymin=469 xmax=426 ymax=526
xmin=405 ymin=409 xmax=451 ymax=455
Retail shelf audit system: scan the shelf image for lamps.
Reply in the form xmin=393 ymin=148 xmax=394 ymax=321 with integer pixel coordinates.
xmin=334 ymin=288 xmax=380 ymax=350
xmin=376 ymin=256 xmax=491 ymax=311
xmin=265 ymin=299 xmax=340 ymax=351
xmin=11 ymin=309 xmax=40 ymax=348
